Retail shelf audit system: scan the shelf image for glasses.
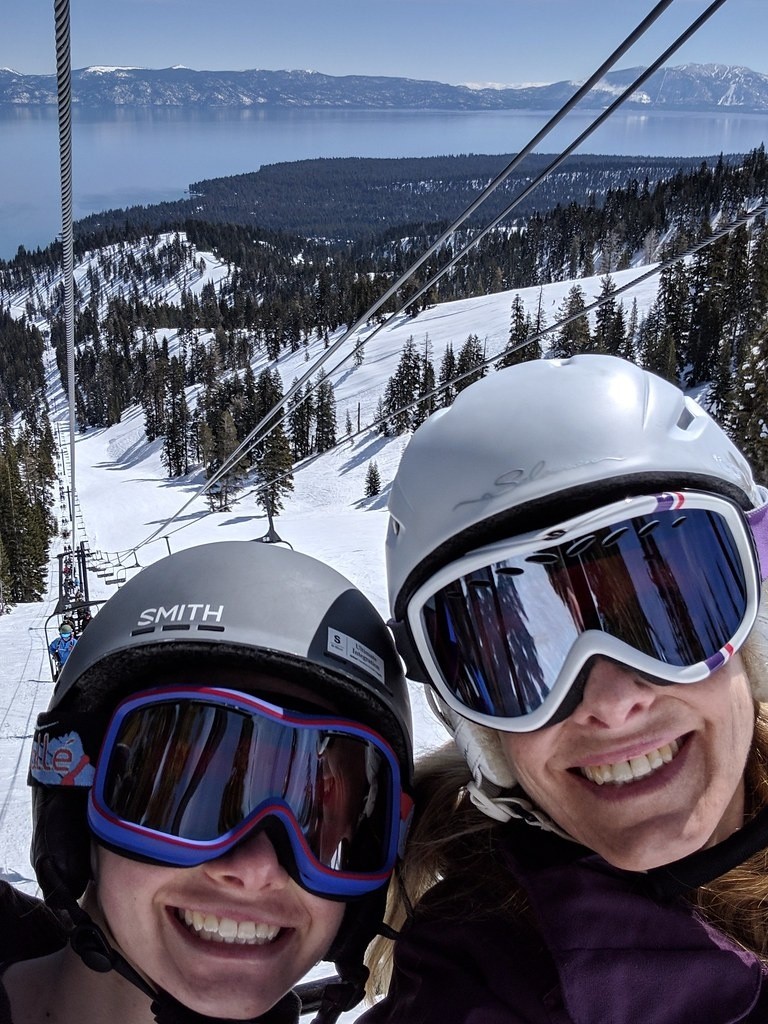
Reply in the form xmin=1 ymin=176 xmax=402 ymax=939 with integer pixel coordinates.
xmin=407 ymin=486 xmax=760 ymax=733
xmin=86 ymin=687 xmax=400 ymax=898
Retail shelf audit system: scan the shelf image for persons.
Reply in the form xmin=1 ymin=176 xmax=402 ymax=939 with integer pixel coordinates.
xmin=0 ymin=354 xmax=768 ymax=1024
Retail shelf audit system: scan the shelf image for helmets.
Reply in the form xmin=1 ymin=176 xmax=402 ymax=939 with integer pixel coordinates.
xmin=27 ymin=542 xmax=414 ymax=973
xmin=385 ymin=354 xmax=768 ymax=795
